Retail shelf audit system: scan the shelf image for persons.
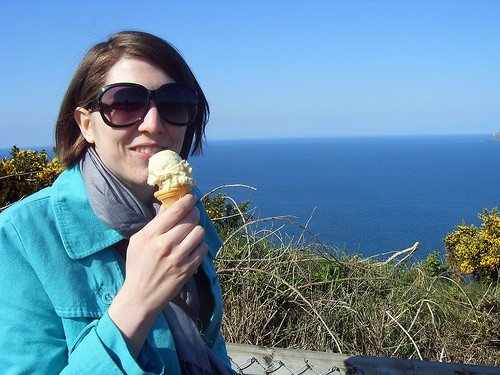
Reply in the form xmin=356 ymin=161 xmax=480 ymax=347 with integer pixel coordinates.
xmin=0 ymin=29 xmax=243 ymax=375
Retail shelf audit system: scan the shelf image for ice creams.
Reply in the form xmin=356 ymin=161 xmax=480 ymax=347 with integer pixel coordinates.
xmin=146 ymin=149 xmax=196 ymax=210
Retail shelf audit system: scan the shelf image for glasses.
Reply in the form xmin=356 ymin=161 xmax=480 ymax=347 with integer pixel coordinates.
xmin=88 ymin=82 xmax=201 ymax=128
